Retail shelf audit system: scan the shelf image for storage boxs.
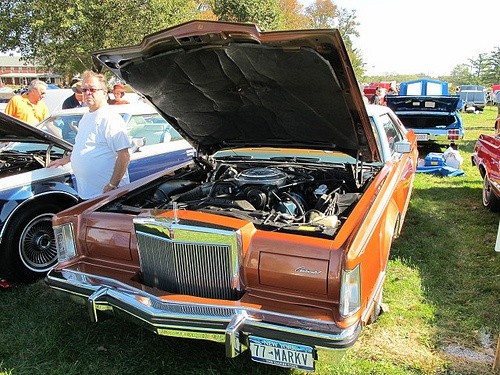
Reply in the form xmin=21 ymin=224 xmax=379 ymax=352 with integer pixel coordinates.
xmin=424 ymin=152 xmax=446 ymax=167
xmin=466 ymin=107 xmax=476 ymax=113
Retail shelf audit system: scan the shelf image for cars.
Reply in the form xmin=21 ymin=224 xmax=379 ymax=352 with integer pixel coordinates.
xmin=383 ymin=79 xmax=464 ymax=147
xmin=0 ymin=19 xmax=420 ymax=371
xmin=471 ymin=134 xmax=500 ymax=210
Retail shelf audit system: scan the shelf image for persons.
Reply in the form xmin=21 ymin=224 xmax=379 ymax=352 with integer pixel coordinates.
xmin=371 ymin=86 xmax=380 ymax=105
xmin=4 ymin=79 xmax=49 ymax=127
xmin=61 ymin=81 xmax=87 ymax=145
xmin=107 ymin=84 xmax=130 ymax=105
xmin=47 ymin=70 xmax=131 ymax=204
xmin=388 ymin=82 xmax=397 ymax=93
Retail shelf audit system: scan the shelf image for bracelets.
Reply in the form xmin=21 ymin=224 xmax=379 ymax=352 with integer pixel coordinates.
xmin=108 ymin=183 xmax=119 ymax=189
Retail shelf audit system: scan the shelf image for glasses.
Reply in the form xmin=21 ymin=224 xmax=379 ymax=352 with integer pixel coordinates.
xmin=81 ymin=88 xmax=104 ymax=93
xmin=35 ymin=88 xmax=46 ymax=95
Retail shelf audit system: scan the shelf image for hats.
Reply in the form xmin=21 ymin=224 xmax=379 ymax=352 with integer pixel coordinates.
xmin=113 ymin=84 xmax=126 ymax=93
xmin=72 ymin=81 xmax=83 ymax=94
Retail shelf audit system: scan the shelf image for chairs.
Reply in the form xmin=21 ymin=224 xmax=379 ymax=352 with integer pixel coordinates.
xmin=134 ymin=124 xmax=164 ymax=145
xmin=456 ymin=99 xmax=462 ymax=113
